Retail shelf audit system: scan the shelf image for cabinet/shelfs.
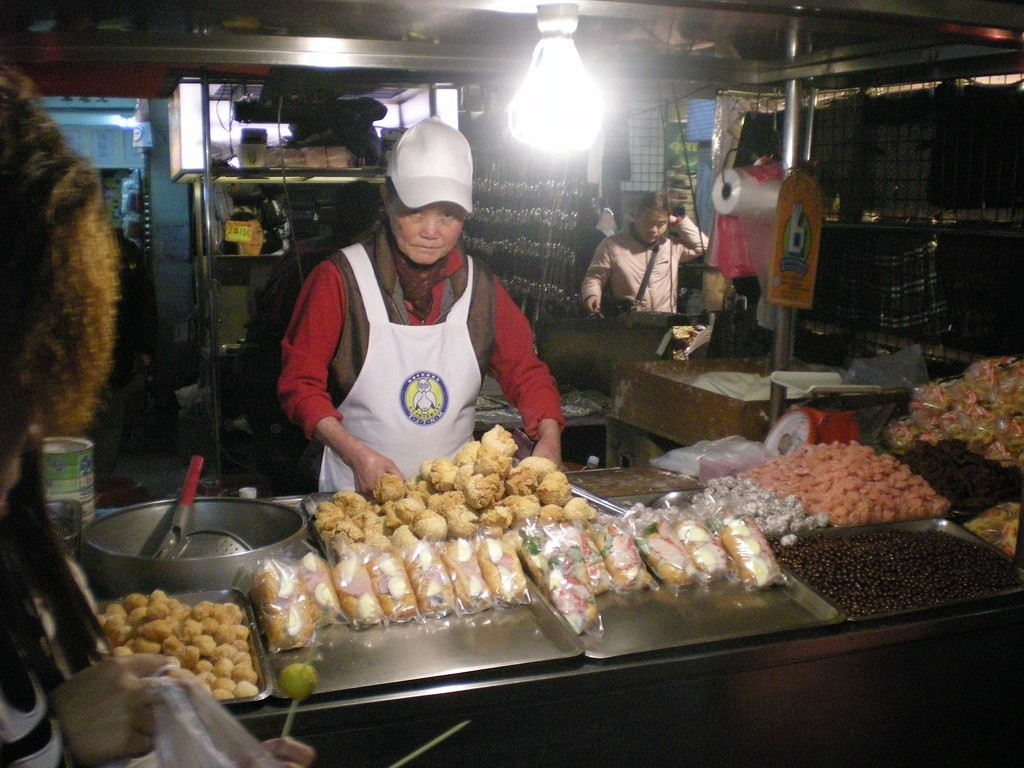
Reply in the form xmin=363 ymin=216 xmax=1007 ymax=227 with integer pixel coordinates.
xmin=173 ymin=60 xmax=488 ymax=492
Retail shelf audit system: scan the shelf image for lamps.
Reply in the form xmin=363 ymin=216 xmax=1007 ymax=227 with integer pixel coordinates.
xmin=507 ymin=4 xmax=605 ymax=151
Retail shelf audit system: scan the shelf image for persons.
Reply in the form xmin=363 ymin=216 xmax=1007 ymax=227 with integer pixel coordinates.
xmin=278 ymin=115 xmax=567 ymax=492
xmin=579 ymin=189 xmax=709 ymax=317
xmin=0 ymin=65 xmax=318 ymax=768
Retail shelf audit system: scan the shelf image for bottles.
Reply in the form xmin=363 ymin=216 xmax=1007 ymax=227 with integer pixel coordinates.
xmin=579 ymin=456 xmax=600 ymax=472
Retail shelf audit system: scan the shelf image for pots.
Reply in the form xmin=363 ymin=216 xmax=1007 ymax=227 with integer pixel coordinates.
xmin=79 ymin=496 xmax=308 ymax=601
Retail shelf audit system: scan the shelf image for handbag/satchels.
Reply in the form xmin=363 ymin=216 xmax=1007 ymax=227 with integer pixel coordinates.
xmin=578 ymin=297 xmax=636 ymax=318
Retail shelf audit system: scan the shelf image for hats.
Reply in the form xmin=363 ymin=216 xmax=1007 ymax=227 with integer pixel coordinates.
xmin=386 ymin=115 xmax=473 ymax=214
